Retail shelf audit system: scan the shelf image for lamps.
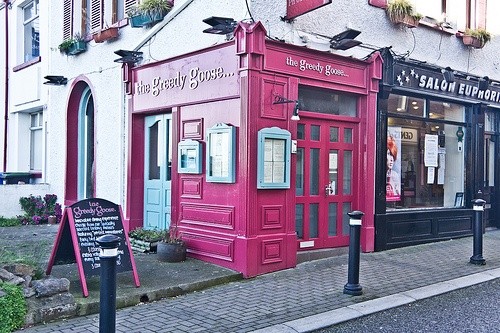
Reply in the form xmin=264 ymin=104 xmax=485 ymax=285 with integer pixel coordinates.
xmin=441 ymin=66 xmax=455 ymax=83
xmin=43 ymin=76 xmax=68 ymax=86
xmin=113 ymin=50 xmax=143 ymax=63
xmin=471 ymin=198 xmax=486 ymax=212
xmin=202 ymin=16 xmax=237 ymax=34
xmin=96 ymin=234 xmax=121 ymax=259
xmin=477 ymin=76 xmax=490 ymax=90
xmin=330 ymin=30 xmax=362 ymax=50
xmin=273 ymin=93 xmax=300 ymax=121
xmin=348 ymin=211 xmax=365 ymax=226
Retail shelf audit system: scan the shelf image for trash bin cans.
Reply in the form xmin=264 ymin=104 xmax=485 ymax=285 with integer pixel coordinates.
xmin=0 ymin=172 xmax=31 ymax=185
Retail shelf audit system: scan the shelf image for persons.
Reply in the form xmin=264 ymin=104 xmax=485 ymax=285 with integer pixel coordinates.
xmin=386 ymin=136 xmax=401 ymax=195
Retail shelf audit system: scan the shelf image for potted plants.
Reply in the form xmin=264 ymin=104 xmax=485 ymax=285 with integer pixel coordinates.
xmin=59 ymin=34 xmax=91 ymax=55
xmin=387 ymin=0 xmax=422 ymax=28
xmin=157 ymin=220 xmax=186 ymax=261
xmin=463 ymin=28 xmax=491 ymax=49
xmin=127 ymin=0 xmax=170 ymax=27
xmin=93 ymin=27 xmax=119 ymax=44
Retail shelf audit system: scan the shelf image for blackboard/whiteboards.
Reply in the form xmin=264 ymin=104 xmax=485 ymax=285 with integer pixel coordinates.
xmin=47 ymin=198 xmax=134 ymax=278
